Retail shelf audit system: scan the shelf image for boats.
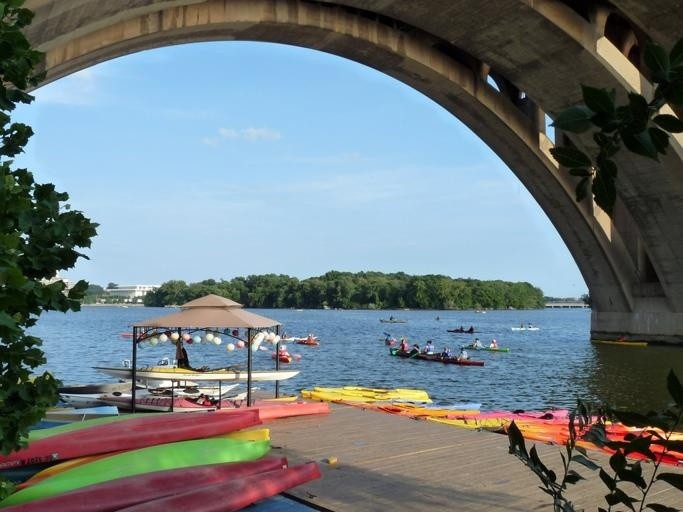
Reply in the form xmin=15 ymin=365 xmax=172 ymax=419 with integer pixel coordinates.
xmin=271 ymin=338 xmax=319 ymax=364
xmin=59 ymin=381 xmax=240 ymax=409
xmin=378 ymin=318 xmax=539 ymax=367
xmin=91 ymin=357 xmax=301 ymax=389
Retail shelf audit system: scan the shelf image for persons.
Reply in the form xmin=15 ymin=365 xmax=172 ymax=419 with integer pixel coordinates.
xmin=528 ymin=323 xmax=533 ymax=328
xmin=385 ymin=333 xmax=497 ymax=361
xmin=454 ymin=326 xmax=464 ymax=332
xmin=469 ymin=326 xmax=473 ymax=332
xmin=390 ymin=315 xmax=393 ymax=320
xmin=282 ymin=332 xmax=287 ymax=339
